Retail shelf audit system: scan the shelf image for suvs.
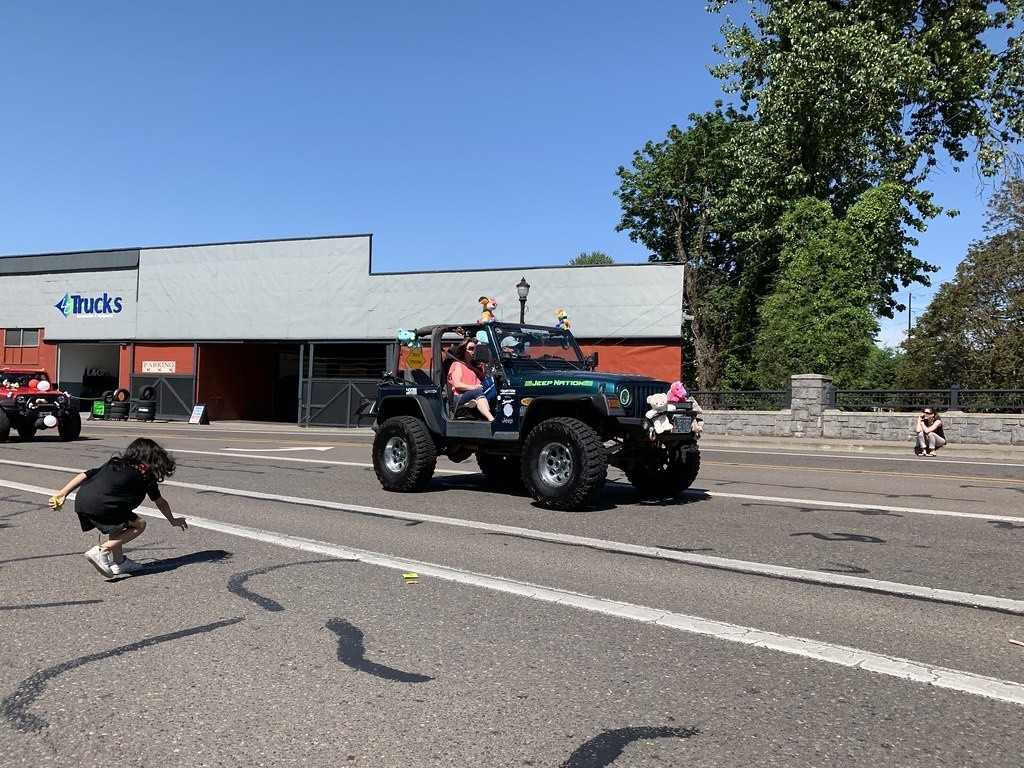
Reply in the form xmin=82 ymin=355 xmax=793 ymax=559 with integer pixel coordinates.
xmin=369 ymin=326 xmax=706 ymax=511
xmin=0 ymin=368 xmax=81 ymax=444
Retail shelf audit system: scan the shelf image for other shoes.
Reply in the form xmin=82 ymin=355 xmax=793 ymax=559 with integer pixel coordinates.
xmin=917 ymin=449 xmax=937 ymax=457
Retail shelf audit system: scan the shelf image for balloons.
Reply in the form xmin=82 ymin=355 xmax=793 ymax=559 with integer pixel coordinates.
xmin=43 ymin=415 xmax=57 ymax=427
xmin=29 ymin=379 xmax=40 ymax=388
xmin=34 ymin=418 xmax=43 ymax=429
xmin=37 ymin=381 xmax=50 ymax=391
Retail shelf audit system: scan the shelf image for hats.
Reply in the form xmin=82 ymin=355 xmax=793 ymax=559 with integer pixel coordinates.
xmin=501 ymin=336 xmax=521 ymax=348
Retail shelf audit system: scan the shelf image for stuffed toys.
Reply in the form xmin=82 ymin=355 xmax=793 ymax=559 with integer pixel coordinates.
xmin=646 ymin=393 xmax=676 ymax=434
xmin=478 ymin=297 xmax=499 ymax=325
xmin=556 ymin=309 xmax=571 ymax=331
xmin=398 ymin=328 xmax=422 ymax=354
xmin=686 ymin=396 xmax=704 ymax=433
xmin=667 ymin=381 xmax=687 ymax=403
xmin=453 ymin=326 xmax=471 ymax=337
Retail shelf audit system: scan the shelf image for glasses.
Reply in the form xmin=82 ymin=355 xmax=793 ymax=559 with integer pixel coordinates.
xmin=923 ymin=411 xmax=932 ymax=415
xmin=465 ymin=347 xmax=475 ymax=350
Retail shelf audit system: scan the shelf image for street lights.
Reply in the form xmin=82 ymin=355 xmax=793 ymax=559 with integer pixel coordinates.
xmin=515 ymin=277 xmax=530 ymax=325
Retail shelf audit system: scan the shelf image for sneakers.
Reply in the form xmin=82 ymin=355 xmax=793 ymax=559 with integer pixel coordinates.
xmin=83 ymin=545 xmax=143 ymax=579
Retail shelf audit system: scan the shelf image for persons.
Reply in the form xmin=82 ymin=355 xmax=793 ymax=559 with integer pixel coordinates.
xmin=501 ymin=336 xmax=522 ymax=355
xmin=916 ymin=407 xmax=947 ymax=457
xmin=447 ymin=338 xmax=495 ymax=422
xmin=48 ymin=437 xmax=188 ymax=578
